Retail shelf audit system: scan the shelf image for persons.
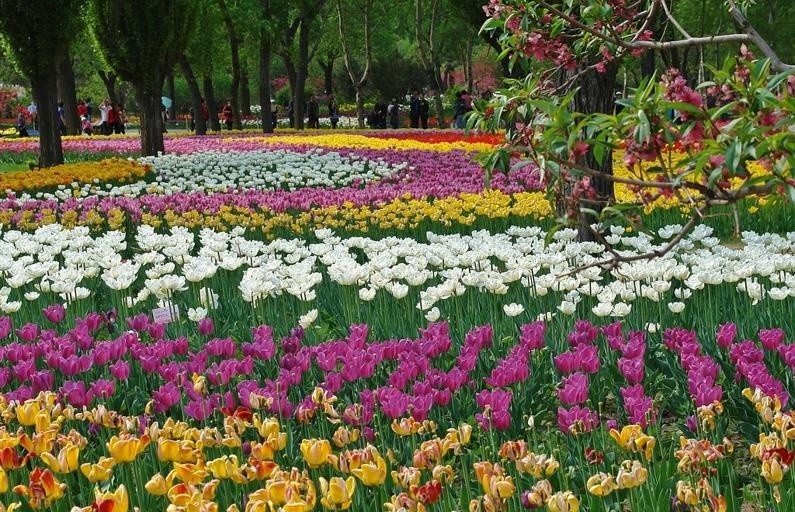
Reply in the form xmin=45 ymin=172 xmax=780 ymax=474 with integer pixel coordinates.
xmin=18 ymin=89 xmax=497 ymax=138
xmin=671 ymin=81 xmax=738 ymax=125
